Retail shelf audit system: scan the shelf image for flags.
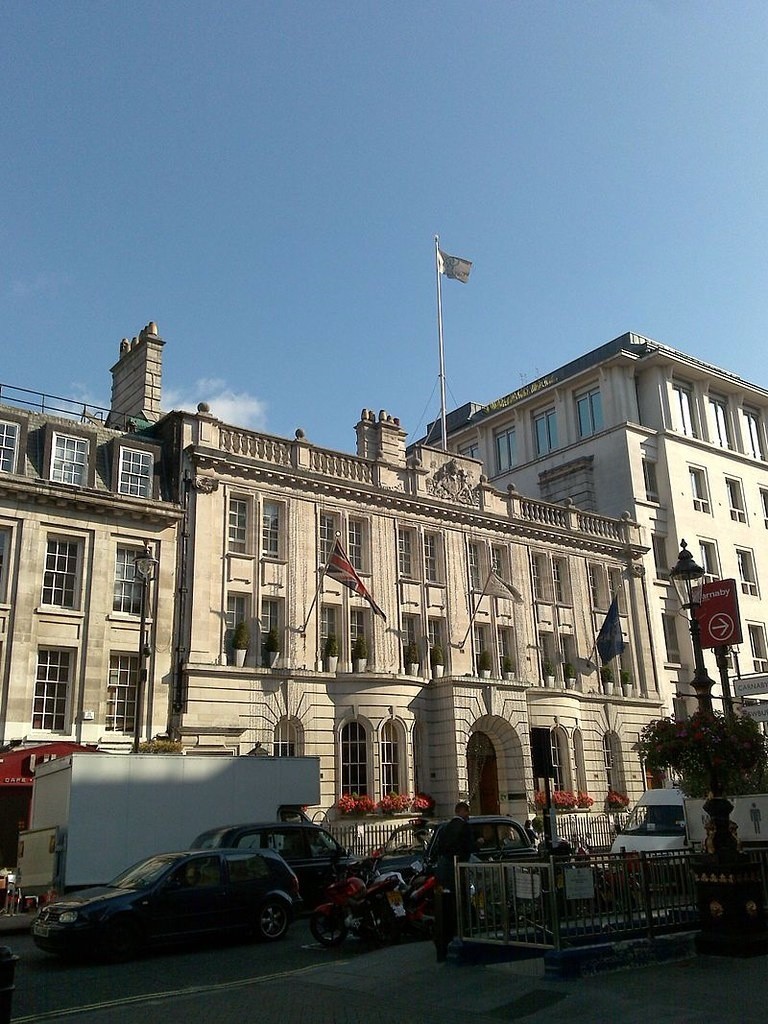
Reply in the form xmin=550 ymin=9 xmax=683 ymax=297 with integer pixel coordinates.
xmin=596 ymin=593 xmax=626 ymax=667
xmin=489 ymin=571 xmax=524 ymax=608
xmin=325 ymin=541 xmax=387 ymax=623
xmin=438 ymin=249 xmax=473 ymax=284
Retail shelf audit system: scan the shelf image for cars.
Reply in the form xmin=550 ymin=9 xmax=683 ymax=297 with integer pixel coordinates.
xmin=29 ymin=847 xmax=303 ymax=960
xmin=188 ymin=822 xmax=361 ymax=910
xmin=371 ymin=813 xmax=542 ymax=881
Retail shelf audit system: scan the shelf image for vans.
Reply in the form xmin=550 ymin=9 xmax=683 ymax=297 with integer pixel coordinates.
xmin=608 ymin=788 xmax=691 ymax=883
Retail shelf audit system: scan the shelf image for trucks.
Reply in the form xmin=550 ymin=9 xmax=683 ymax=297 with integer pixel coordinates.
xmin=14 ymin=751 xmax=326 ymax=902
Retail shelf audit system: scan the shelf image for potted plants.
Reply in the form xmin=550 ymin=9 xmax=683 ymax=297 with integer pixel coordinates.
xmin=503 ymin=652 xmax=516 ymax=681
xmin=619 ymin=668 xmax=633 ymax=697
xmin=479 ymin=650 xmax=492 ymax=678
xmin=265 ymin=625 xmax=281 ymax=668
xmin=565 ymin=663 xmax=577 ymax=690
xmin=600 ymin=665 xmax=615 ymax=696
xmin=353 ymin=635 xmax=368 ymax=672
xmin=430 ymin=645 xmax=445 ymax=678
xmin=324 ymin=631 xmax=338 ymax=672
xmin=544 ymin=658 xmax=556 ymax=688
xmin=406 ymin=641 xmax=420 ymax=676
xmin=231 ymin=620 xmax=250 ymax=666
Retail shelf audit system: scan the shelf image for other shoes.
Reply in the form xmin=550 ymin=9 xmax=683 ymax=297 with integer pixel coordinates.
xmin=436 ymin=950 xmax=448 ymax=963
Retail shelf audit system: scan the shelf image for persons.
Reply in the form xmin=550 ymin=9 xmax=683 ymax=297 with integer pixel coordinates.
xmin=524 ymin=820 xmax=539 ymax=846
xmin=426 ymin=802 xmax=484 ymax=951
xmin=505 ymin=814 xmax=515 ymax=840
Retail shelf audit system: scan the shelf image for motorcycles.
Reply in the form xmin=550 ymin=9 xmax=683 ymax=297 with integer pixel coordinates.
xmin=307 ymin=845 xmax=438 ymax=949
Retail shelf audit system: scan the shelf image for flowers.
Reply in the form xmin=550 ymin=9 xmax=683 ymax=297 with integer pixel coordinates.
xmin=413 ymin=794 xmax=433 ymax=811
xmin=634 ymin=711 xmax=764 ymax=798
xmin=606 ymin=791 xmax=630 ymax=810
xmin=577 ymin=793 xmax=594 ymax=808
xmin=339 ymin=793 xmax=377 ymax=815
xmin=536 ymin=790 xmax=577 ymax=808
xmin=376 ymin=791 xmax=412 ymax=813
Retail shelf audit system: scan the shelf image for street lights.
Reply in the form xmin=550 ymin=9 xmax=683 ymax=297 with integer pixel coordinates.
xmin=130 ymin=547 xmax=161 ymax=754
xmin=668 ymin=538 xmax=768 ymax=959
